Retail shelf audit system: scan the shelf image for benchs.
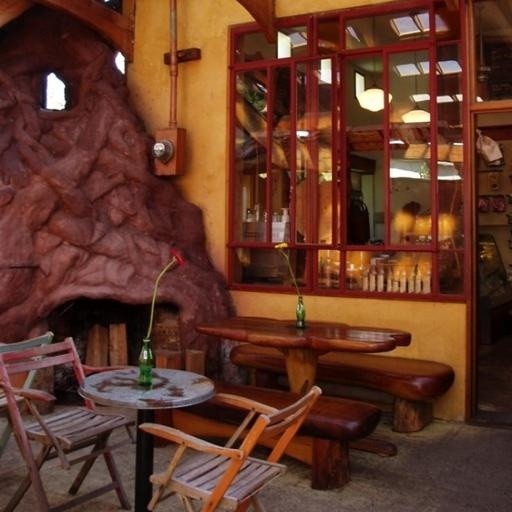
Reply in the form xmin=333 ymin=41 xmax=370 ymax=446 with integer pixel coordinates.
xmin=227 ymin=341 xmax=454 ymax=436
xmin=162 ymin=379 xmax=383 ymax=489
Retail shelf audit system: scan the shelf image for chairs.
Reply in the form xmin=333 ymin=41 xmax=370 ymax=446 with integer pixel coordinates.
xmin=0 ymin=330 xmax=131 ymax=511
xmin=139 ymin=383 xmax=322 ymax=512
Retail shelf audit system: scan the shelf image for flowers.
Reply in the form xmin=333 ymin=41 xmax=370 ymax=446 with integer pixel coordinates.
xmin=274 ymin=239 xmax=305 ymax=302
xmin=138 ymin=244 xmax=187 ymax=344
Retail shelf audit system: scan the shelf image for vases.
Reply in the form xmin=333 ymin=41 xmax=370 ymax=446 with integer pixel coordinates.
xmin=135 ymin=335 xmax=156 ymax=386
xmin=296 ymin=296 xmax=306 ymax=328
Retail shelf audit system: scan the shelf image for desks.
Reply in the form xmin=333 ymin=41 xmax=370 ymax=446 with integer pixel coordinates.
xmin=76 ymin=363 xmax=217 ymax=512
xmin=193 ymin=316 xmax=413 ymax=458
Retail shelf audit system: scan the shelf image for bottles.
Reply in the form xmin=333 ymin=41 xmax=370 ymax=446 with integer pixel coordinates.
xmin=138 ymin=338 xmax=154 ymax=387
xmin=241 ymin=208 xmax=291 ymax=243
xmin=295 ymin=295 xmax=306 ymax=330
xmin=322 ymin=259 xmax=360 ymax=292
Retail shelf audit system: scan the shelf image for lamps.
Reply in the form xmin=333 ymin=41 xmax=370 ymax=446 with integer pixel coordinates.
xmin=357 ymin=14 xmax=393 ymax=111
